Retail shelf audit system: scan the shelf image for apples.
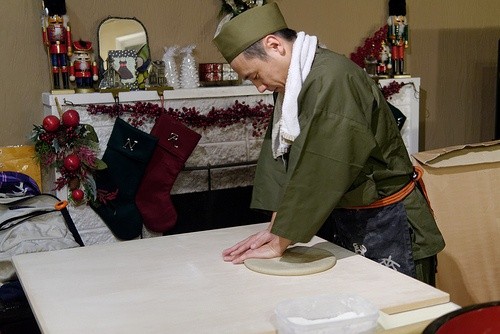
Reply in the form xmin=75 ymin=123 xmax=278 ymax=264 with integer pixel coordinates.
xmin=64 ymin=154 xmax=77 ymax=170
xmin=73 ymin=189 xmax=85 ymax=201
xmin=62 ymin=110 xmax=78 ymax=126
xmin=43 ymin=115 xmax=58 ymax=131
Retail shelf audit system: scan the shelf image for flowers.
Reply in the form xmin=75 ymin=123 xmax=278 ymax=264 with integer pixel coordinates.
xmin=25 ymin=97 xmax=107 ymax=207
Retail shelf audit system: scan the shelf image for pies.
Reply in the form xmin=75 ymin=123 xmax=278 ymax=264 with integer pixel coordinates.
xmin=244 ymin=245 xmax=336 ymax=275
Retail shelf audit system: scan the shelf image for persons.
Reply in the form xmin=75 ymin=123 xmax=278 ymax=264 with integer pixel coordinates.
xmin=69 ymin=40 xmax=98 ymax=90
xmin=41 ymin=0 xmax=72 ymax=90
xmin=376 ymin=40 xmax=391 ymax=73
xmin=387 ymin=0 xmax=408 ymax=75
xmin=215 ymin=1 xmax=448 ymax=334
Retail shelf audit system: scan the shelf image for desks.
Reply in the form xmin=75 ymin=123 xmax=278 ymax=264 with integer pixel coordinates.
xmin=12 ymin=224 xmax=462 ymax=334
xmin=42 ymin=77 xmax=420 ymax=200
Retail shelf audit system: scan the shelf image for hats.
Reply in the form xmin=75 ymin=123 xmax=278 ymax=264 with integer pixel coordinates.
xmin=212 ymin=1 xmax=287 ymax=65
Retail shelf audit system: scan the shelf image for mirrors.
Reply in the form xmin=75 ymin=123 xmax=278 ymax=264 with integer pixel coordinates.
xmin=97 ymin=15 xmax=149 ymax=60
